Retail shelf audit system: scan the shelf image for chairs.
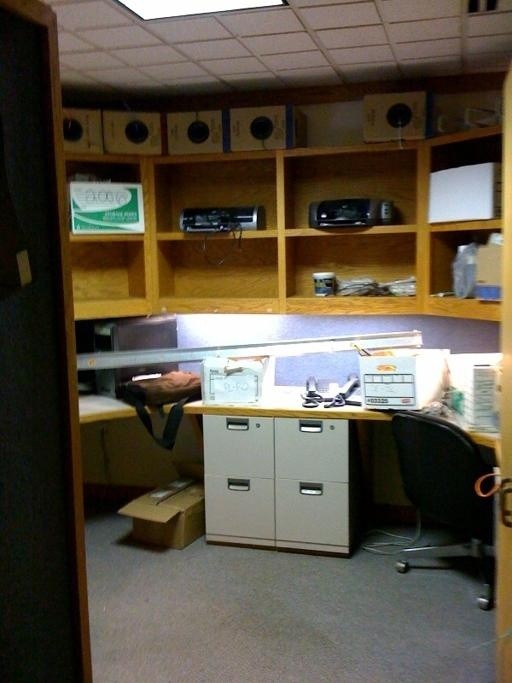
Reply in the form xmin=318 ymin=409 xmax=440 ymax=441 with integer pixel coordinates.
xmin=392 ymin=406 xmax=498 ymax=611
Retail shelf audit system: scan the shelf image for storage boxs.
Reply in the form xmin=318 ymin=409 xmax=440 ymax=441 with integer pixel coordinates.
xmin=68 ymin=179 xmax=147 ymax=237
xmin=62 ymin=103 xmax=308 ymax=156
xmin=359 ymin=347 xmax=450 ymax=411
xmin=201 ymin=355 xmax=277 ymax=406
xmin=425 ymin=161 xmax=502 ymax=224
xmin=363 ymin=90 xmax=429 ymax=145
xmin=116 ymin=461 xmax=205 ymax=551
xmin=474 ymin=250 xmax=503 ymax=303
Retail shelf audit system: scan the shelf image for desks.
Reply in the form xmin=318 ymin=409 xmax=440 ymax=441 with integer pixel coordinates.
xmin=74 ymin=389 xmax=499 ymax=455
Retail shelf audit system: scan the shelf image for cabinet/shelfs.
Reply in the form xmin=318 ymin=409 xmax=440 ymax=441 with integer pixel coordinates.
xmin=199 ymin=413 xmax=373 ymax=558
xmin=63 ymin=126 xmax=503 ymax=322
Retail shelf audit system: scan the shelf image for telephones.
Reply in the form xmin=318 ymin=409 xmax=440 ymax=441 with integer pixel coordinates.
xmin=306 ymin=375 xmax=362 ymax=406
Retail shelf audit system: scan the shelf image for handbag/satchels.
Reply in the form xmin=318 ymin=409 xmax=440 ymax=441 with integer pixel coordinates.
xmin=120 ymin=370 xmax=203 ymax=406
